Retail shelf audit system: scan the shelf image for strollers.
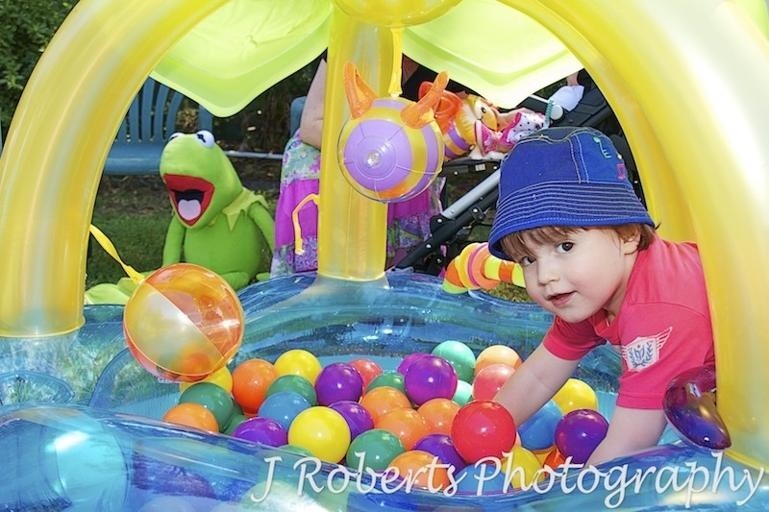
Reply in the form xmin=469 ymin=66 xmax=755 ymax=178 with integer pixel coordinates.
xmin=277 ymin=83 xmax=624 ymax=353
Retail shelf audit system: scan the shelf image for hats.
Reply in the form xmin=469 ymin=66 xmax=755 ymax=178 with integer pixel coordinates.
xmin=488 ymin=125 xmax=658 ymax=263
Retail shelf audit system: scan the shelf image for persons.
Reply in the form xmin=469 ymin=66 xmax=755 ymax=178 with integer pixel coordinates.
xmin=0 ymin=0 xmax=769 ymax=512
